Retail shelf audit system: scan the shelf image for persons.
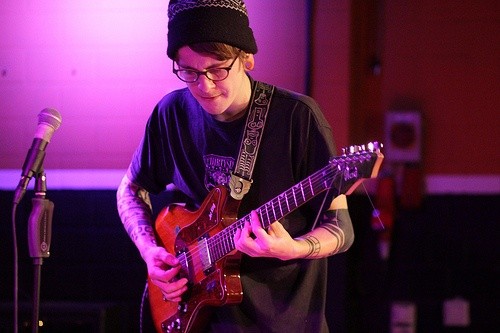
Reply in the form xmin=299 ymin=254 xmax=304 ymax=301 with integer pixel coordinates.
xmin=116 ymin=0 xmax=355 ymax=333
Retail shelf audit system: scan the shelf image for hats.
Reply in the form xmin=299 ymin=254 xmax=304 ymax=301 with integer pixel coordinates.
xmin=167 ymin=0 xmax=258 ymax=61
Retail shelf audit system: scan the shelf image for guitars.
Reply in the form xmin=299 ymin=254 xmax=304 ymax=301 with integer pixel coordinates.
xmin=147 ymin=142 xmax=385 ymax=333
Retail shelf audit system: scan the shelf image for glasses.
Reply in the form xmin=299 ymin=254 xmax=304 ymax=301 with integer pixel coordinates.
xmin=172 ymin=49 xmax=241 ymax=84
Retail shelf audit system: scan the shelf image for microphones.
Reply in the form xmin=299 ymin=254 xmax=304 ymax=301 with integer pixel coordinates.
xmin=14 ymin=108 xmax=61 ymax=205
